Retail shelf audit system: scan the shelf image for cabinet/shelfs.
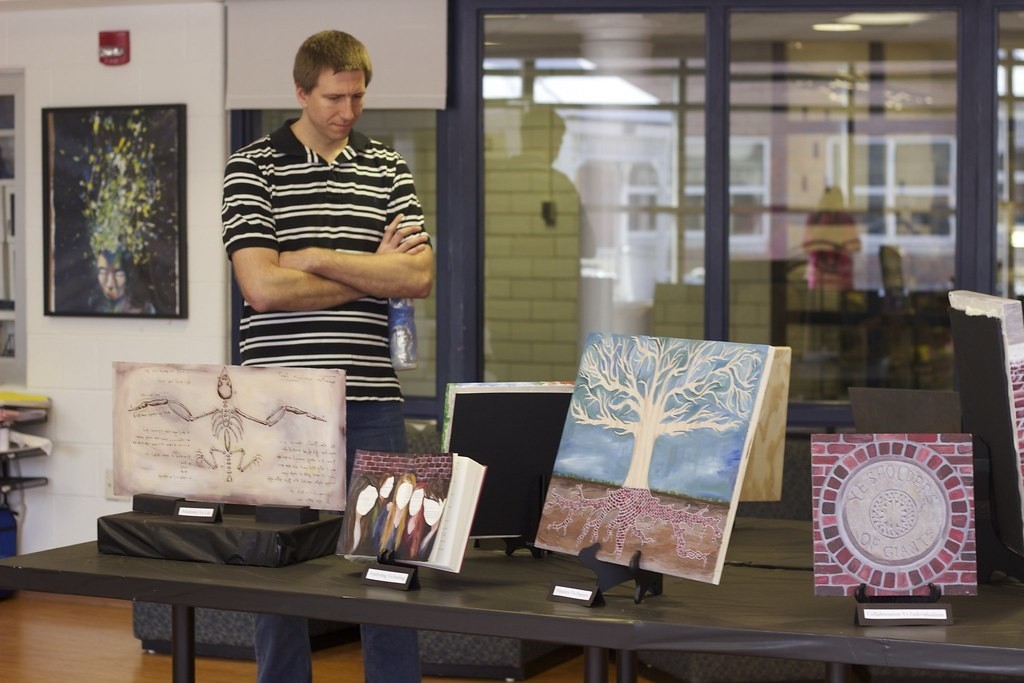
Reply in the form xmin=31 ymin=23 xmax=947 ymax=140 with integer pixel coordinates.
xmin=0 ymin=70 xmax=25 ymax=383
xmin=0 ymin=405 xmax=49 ymax=493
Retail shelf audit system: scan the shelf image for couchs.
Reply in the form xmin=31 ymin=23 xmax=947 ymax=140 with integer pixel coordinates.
xmin=634 ymin=440 xmax=828 ymax=683
xmin=416 ymin=429 xmax=583 ymax=681
xmin=132 ymin=424 xmax=422 ymax=661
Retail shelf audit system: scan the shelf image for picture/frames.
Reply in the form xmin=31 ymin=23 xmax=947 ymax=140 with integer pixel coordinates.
xmin=40 ymin=104 xmax=189 ymax=320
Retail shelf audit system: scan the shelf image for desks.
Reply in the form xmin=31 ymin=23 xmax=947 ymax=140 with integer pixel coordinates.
xmin=0 ymin=516 xmax=1024 ymax=682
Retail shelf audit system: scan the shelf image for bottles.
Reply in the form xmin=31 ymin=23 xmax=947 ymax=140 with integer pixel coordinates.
xmin=387 ymin=298 xmax=418 ymax=371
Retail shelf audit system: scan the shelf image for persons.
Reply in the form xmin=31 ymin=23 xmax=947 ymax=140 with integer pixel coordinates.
xmin=484 ymin=108 xmax=595 ymax=383
xmin=221 ymin=30 xmax=434 ymax=683
xmin=801 ymin=186 xmax=862 ymax=365
